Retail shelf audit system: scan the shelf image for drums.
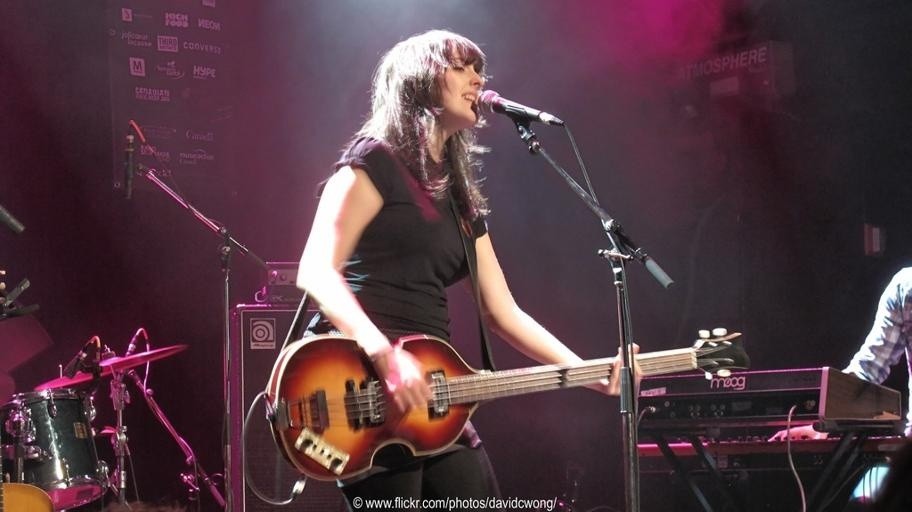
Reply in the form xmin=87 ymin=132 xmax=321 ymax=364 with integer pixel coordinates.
xmin=3 ymin=389 xmax=107 ymax=508
xmin=0 ymin=483 xmax=55 ymax=511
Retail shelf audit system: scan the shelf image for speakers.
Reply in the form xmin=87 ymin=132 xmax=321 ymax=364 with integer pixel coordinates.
xmin=225 ymin=303 xmax=355 ymax=512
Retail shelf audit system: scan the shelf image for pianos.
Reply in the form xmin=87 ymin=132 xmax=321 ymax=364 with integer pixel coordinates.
xmin=635 ymin=438 xmax=906 ymax=459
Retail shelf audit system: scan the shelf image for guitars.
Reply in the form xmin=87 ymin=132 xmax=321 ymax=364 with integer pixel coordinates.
xmin=263 ymin=326 xmax=747 ymax=488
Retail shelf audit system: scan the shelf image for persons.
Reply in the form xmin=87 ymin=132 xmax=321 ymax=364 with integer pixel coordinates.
xmin=295 ymin=28 xmax=642 ymax=512
xmin=762 ymin=261 xmax=911 ymax=511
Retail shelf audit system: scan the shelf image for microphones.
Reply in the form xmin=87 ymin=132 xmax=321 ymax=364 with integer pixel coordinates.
xmin=478 ymin=89 xmax=567 ymax=127
xmin=123 ymin=135 xmax=135 ymax=200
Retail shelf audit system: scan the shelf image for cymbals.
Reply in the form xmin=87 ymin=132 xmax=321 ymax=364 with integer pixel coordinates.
xmin=34 ymin=341 xmax=187 ymax=390
